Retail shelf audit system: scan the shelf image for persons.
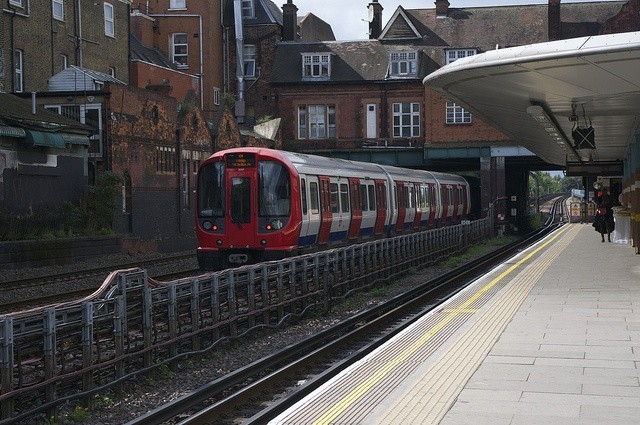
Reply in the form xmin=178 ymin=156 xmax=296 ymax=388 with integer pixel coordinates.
xmin=591 ymin=186 xmax=616 ymax=244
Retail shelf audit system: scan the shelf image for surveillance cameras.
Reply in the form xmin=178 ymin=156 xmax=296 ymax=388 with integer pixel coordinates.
xmin=569 ymin=103 xmax=579 ymax=121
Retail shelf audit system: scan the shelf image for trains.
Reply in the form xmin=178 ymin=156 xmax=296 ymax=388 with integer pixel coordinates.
xmin=192 ymin=145 xmax=480 ymax=270
